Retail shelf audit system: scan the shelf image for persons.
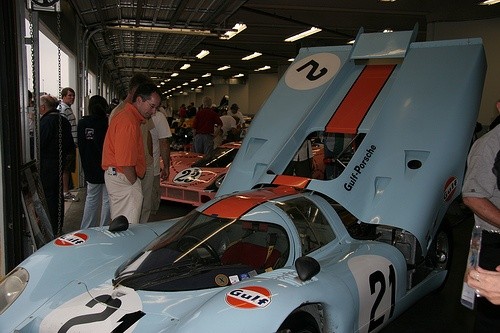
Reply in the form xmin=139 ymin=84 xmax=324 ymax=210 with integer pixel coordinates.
xmin=31 ymin=95 xmax=75 ymax=239
xmin=284 ymin=138 xmax=314 ymax=180
xmin=108 ymin=73 xmax=173 ymax=224
xmin=25 ymin=88 xmax=81 ymax=202
xmin=101 ymin=82 xmax=161 ymax=224
xmin=77 ymin=95 xmax=111 ymax=229
xmin=463 ymin=100 xmax=500 ymax=333
xmin=161 ymin=96 xmax=247 ymax=162
xmin=465 ymin=265 xmax=500 ymax=305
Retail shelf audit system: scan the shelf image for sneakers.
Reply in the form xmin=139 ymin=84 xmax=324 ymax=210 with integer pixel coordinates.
xmin=64 ymin=192 xmax=80 ymax=202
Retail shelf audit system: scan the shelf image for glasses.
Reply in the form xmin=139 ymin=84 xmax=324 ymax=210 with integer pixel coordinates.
xmin=146 ymin=101 xmax=160 ymax=110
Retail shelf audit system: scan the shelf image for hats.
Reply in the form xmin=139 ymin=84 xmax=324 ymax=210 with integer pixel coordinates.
xmin=230 ymin=104 xmax=239 ymax=110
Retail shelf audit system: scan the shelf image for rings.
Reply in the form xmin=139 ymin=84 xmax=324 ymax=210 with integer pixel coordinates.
xmin=475 ymin=289 xmax=480 ymax=297
xmin=473 ymin=272 xmax=480 ymax=280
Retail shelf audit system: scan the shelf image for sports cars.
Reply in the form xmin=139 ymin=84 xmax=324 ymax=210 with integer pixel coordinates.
xmin=0 ymin=23 xmax=488 ymax=333
xmin=160 ymin=140 xmax=242 ymax=208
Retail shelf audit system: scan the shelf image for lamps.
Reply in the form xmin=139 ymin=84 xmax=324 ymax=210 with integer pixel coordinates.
xmin=196 ymin=8 xmax=322 ymax=61
